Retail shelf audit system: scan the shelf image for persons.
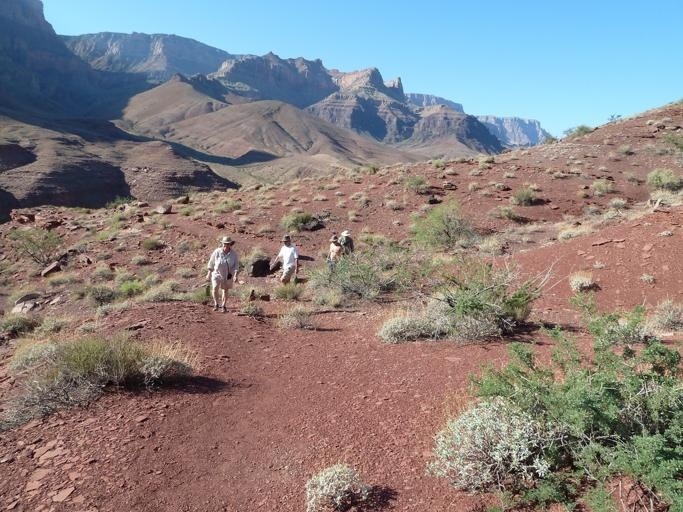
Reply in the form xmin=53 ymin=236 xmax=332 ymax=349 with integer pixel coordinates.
xmin=273 ymin=235 xmax=298 ymax=284
xmin=337 ymin=229 xmax=354 ymax=259
xmin=205 ymin=235 xmax=241 ymax=313
xmin=325 ymin=235 xmax=343 ymax=272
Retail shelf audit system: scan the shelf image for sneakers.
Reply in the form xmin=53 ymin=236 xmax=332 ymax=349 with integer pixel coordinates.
xmin=213 ymin=304 xmax=227 ymax=313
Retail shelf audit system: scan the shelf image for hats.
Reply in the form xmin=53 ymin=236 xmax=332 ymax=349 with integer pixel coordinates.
xmin=329 ymin=235 xmax=338 ymax=242
xmin=341 ymin=230 xmax=351 ymax=237
xmin=220 ymin=236 xmax=234 ymax=246
xmin=281 ymin=235 xmax=291 ymax=242
